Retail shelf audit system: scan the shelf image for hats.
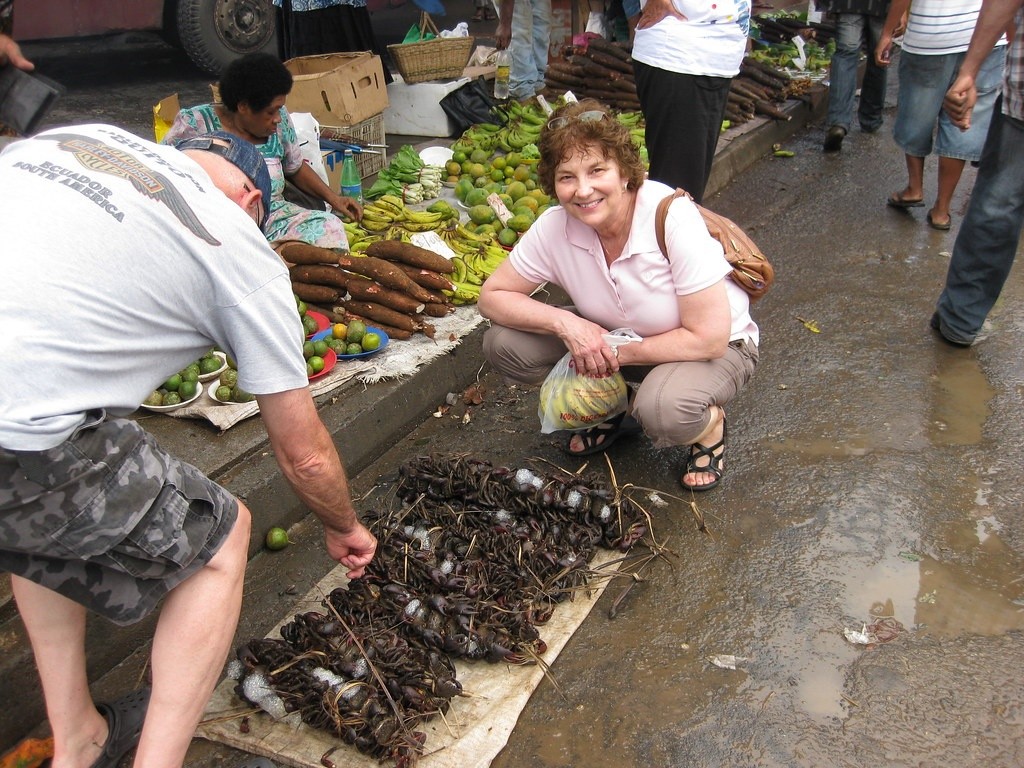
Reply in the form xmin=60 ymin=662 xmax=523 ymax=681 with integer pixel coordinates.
xmin=175 ymin=131 xmax=272 ymax=237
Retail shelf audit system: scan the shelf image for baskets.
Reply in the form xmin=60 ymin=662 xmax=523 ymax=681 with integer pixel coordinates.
xmin=388 ymin=11 xmax=474 ymax=84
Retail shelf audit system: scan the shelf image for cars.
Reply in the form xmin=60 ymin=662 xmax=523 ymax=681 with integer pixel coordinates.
xmin=1 ymin=0 xmax=282 ymax=83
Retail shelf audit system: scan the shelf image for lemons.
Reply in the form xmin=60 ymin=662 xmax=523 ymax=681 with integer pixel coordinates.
xmin=266 ymin=527 xmax=290 ymax=550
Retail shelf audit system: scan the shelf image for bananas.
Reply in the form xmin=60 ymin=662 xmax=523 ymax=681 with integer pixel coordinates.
xmin=451 ymin=98 xmax=650 ymax=172
xmin=540 ymin=370 xmax=627 ymax=429
xmin=719 ymin=120 xmax=730 ymax=133
xmin=343 ymin=194 xmax=513 ymax=305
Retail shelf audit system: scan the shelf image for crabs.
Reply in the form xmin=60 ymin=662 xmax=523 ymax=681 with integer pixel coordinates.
xmin=236 ymin=448 xmax=651 ymax=768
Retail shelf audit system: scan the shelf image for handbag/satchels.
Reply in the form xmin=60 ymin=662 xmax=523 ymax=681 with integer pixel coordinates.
xmin=538 ymin=328 xmax=642 ymax=434
xmin=439 ymin=75 xmax=508 ymax=139
xmin=656 ymin=188 xmax=773 ymax=304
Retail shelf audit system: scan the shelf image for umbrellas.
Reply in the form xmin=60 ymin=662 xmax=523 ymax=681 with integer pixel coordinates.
xmin=314 ymin=124 xmax=390 ymax=155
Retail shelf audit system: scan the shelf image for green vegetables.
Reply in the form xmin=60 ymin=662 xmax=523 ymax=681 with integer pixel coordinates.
xmin=747 ymin=9 xmax=837 ymax=71
xmin=364 ymin=144 xmax=446 ymax=204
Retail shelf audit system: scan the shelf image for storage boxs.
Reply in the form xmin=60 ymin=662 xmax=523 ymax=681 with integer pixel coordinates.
xmin=209 ymin=48 xmax=471 ymax=196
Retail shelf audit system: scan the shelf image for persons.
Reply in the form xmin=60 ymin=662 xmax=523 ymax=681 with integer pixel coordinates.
xmin=873 ymin=0 xmax=1011 ymax=230
xmin=812 ymin=0 xmax=908 ymax=155
xmin=271 ymin=1 xmax=395 ymax=86
xmin=930 ymin=0 xmax=1024 ymax=347
xmin=630 ymin=0 xmax=752 ymax=205
xmin=0 ymin=128 xmax=379 ymax=768
xmin=491 ymin=0 xmax=555 ymax=104
xmin=468 ymin=0 xmax=497 ymax=21
xmin=474 ymin=99 xmax=759 ymax=491
xmin=159 ymin=50 xmax=365 ymax=256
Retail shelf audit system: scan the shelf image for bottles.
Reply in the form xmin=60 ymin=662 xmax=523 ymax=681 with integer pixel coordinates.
xmin=340 ymin=150 xmax=363 ymax=222
xmin=494 ymin=47 xmax=512 ymax=100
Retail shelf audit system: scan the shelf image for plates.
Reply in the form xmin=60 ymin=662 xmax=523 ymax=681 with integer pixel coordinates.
xmin=141 ymin=310 xmax=389 ymax=413
xmin=456 ymin=199 xmax=470 ymax=209
xmin=441 ymin=181 xmax=457 ymax=187
xmin=418 ymin=146 xmax=455 ymax=168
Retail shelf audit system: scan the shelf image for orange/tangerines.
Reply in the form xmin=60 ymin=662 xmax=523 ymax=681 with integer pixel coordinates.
xmin=147 ymin=292 xmax=380 ymax=406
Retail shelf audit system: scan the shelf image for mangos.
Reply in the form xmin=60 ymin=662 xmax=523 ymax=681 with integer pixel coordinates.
xmin=428 ymin=149 xmax=560 ymax=247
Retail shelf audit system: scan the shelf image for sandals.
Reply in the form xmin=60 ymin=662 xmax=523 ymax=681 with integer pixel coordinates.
xmin=681 ymin=405 xmax=728 ymax=491
xmin=567 ymin=383 xmax=636 ymax=456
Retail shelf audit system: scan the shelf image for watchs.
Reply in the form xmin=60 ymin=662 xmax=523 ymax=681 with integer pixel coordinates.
xmin=610 ymin=342 xmax=618 ymax=359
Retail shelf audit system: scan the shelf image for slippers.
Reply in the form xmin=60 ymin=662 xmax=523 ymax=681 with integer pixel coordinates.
xmin=87 ymin=686 xmax=150 ymax=768
xmin=888 ymin=189 xmax=926 ymax=207
xmin=927 ymin=207 xmax=952 ymax=229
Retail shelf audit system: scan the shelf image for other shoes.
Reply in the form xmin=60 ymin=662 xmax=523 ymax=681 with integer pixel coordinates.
xmin=537 ymin=86 xmax=558 ymax=102
xmin=520 ymin=95 xmax=541 ymax=112
xmin=823 ymin=125 xmax=845 ymax=151
